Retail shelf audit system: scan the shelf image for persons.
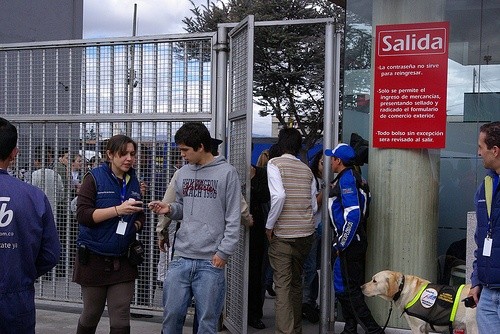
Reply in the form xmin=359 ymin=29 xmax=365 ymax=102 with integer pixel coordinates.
xmin=267 ymin=127 xmax=319 ymax=334
xmin=256 ymin=148 xmax=276 ymax=298
xmin=6 ymin=139 xmax=108 ymax=283
xmin=269 ymin=142 xmax=282 ymax=160
xmin=468 ymin=120 xmax=500 ymax=334
xmin=77 ymin=135 xmax=146 ymax=334
xmin=301 ymin=149 xmax=333 ymax=321
xmin=325 ymin=142 xmax=384 ymax=334
xmin=0 ymin=117 xmax=59 ymax=334
xmin=146 ymin=124 xmax=240 ymax=334
xmin=157 ymin=137 xmax=254 ymax=334
xmin=246 ymin=142 xmax=267 ymax=329
xmin=126 ymin=142 xmax=188 ymax=318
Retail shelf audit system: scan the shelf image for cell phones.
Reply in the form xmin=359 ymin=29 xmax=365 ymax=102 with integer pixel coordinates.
xmin=462 ymin=294 xmax=482 ymax=308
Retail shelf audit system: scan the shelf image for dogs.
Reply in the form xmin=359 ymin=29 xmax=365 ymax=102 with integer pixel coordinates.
xmin=359 ymin=271 xmax=479 ymax=334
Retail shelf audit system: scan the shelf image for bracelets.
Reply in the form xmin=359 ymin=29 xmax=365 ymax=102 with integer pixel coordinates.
xmin=168 ymin=203 xmax=173 ymax=214
xmin=115 ymin=206 xmax=119 ymax=217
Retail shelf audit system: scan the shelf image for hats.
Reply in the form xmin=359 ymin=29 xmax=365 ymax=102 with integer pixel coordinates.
xmin=325 ymin=143 xmax=355 ymax=163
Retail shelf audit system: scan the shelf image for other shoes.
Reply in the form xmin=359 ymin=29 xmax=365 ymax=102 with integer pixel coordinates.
xmin=265 ymin=284 xmax=276 ymax=296
xmin=301 ymin=303 xmax=320 ymax=323
xmin=132 ymin=312 xmax=153 ymax=318
xmin=249 ymin=320 xmax=266 ymax=329
